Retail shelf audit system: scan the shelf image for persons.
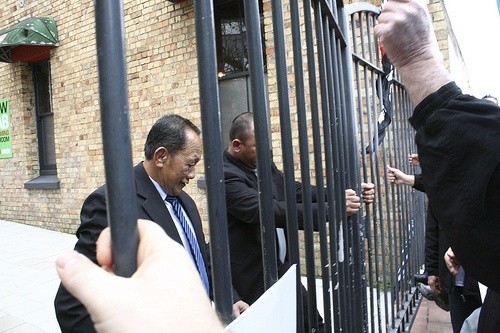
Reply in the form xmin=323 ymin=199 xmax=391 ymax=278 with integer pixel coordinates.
xmin=56 ymin=218 xmax=227 ymax=333
xmin=54 ymin=115 xmax=249 ymax=333
xmin=223 ymin=112 xmax=375 ymax=333
xmin=375 ymin=0 xmax=500 ymax=333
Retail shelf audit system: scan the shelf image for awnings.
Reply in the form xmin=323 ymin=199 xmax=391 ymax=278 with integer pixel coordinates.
xmin=0 ymin=16 xmax=60 ymax=64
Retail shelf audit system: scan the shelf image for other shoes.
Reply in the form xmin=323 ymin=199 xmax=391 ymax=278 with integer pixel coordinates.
xmin=405 ymin=273 xmax=428 ymax=287
xmin=416 ymin=281 xmax=449 ymax=312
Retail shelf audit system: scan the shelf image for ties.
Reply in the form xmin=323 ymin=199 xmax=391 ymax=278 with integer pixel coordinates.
xmin=251 ymin=168 xmax=286 ymax=264
xmin=165 ymin=194 xmax=210 ymax=298
